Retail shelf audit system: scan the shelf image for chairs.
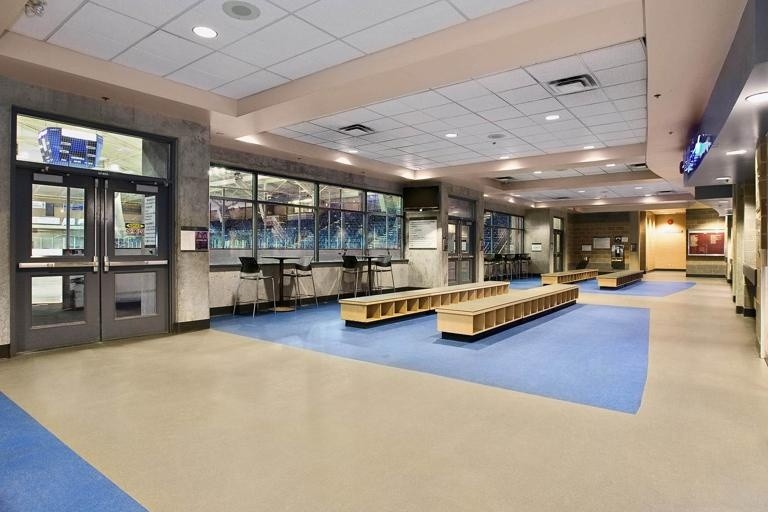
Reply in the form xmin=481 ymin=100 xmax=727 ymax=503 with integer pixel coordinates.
xmin=233 ymin=255 xmax=396 ymax=319
xmin=484 ymin=253 xmax=531 ymax=281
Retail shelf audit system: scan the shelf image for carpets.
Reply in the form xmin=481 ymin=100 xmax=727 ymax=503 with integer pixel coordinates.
xmin=0 ymin=391 xmax=147 ymax=512
xmin=210 ymin=294 xmax=650 ymax=415
xmin=485 ymin=277 xmax=696 ymax=297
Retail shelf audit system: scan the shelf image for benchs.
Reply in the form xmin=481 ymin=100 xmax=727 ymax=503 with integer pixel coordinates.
xmin=686 ymin=261 xmax=727 ymax=278
